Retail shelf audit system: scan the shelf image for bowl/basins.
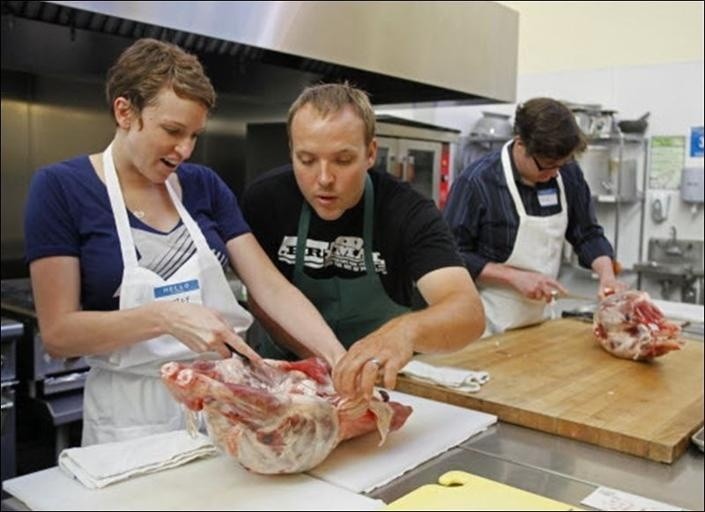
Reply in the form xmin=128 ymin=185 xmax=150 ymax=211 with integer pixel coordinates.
xmin=474 ymin=110 xmax=515 ymax=137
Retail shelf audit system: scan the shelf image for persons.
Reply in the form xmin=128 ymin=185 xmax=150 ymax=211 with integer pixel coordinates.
xmin=232 ymin=80 xmax=490 ymax=410
xmin=437 ymin=95 xmax=625 ymax=344
xmin=18 ymin=32 xmax=349 ymax=452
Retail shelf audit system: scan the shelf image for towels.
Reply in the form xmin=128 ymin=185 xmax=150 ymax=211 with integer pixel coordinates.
xmin=392 ymin=357 xmax=495 ymax=396
xmin=57 ymin=423 xmax=218 ymax=492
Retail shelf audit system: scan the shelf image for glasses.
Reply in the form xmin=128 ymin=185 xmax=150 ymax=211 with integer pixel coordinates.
xmin=531 ymin=154 xmax=576 ymax=172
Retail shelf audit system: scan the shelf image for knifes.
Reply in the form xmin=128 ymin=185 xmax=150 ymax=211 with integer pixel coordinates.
xmin=547 ymin=291 xmax=597 ymax=304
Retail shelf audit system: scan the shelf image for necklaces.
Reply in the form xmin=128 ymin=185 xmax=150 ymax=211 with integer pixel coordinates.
xmin=117 ymin=180 xmax=166 ymax=224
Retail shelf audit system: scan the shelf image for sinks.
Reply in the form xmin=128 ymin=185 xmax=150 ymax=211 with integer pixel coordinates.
xmin=632 ymin=260 xmax=688 ymax=280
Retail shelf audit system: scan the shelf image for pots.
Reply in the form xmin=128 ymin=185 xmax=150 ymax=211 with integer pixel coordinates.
xmin=618 ymin=111 xmax=654 ymax=135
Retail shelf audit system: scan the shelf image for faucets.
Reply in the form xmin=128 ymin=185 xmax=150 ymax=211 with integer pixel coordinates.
xmin=670 ymin=224 xmax=676 ymax=245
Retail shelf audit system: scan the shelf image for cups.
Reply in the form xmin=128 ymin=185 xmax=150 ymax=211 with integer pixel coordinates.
xmin=571 ymin=108 xmax=617 ymax=134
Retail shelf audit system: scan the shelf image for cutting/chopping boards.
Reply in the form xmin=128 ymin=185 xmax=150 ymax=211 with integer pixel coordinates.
xmin=386 ymin=313 xmax=703 ymax=466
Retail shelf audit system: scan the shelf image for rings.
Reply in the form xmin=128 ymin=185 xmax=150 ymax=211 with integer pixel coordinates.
xmin=369 ymin=358 xmax=380 ymax=368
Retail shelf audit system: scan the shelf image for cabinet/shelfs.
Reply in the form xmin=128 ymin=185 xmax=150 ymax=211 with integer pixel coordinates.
xmin=463 ymin=133 xmax=647 ymax=311
xmin=243 ymin=119 xmax=462 ymax=210
